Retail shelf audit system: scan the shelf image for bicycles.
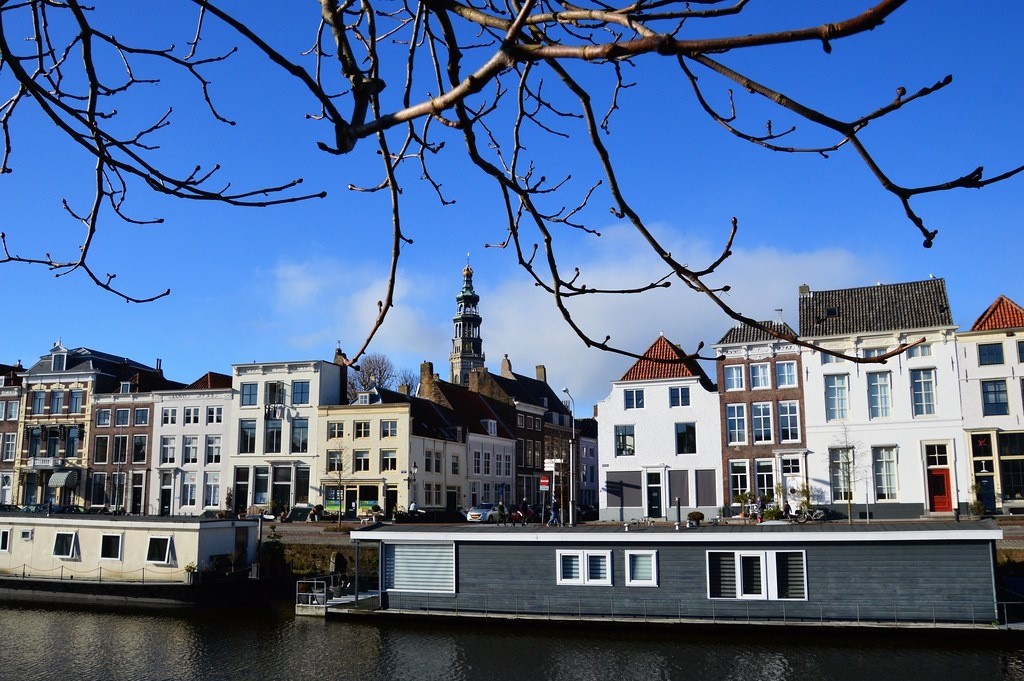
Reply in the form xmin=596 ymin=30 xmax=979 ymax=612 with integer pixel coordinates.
xmin=796 ymin=502 xmax=827 ymax=524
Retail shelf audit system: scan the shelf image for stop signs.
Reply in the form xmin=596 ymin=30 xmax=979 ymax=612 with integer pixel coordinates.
xmin=540 ymin=477 xmax=550 ymax=485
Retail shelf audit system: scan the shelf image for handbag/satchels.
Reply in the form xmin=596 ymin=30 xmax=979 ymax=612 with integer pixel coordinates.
xmin=783 ymin=512 xmax=785 ymax=516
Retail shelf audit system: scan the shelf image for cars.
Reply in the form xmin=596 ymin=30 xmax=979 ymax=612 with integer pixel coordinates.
xmin=0 ymin=504 xmax=21 ymax=512
xmin=509 ymin=504 xmax=599 ymax=524
xmin=20 ymin=505 xmax=108 ymax=516
xmin=466 ymin=502 xmax=510 ymax=524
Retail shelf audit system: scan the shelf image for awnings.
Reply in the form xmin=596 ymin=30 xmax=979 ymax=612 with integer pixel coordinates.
xmin=48 ymin=470 xmax=77 ymax=489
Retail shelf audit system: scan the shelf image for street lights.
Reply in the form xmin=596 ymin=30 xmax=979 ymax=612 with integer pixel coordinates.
xmin=562 ymin=387 xmax=581 ymax=528
xmin=95 ymin=408 xmax=123 ymax=516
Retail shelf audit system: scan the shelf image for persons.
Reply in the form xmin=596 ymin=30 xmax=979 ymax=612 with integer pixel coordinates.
xmin=783 ymin=500 xmax=792 ymax=522
xmin=546 ymin=499 xmax=561 ymax=527
xmin=497 ymin=501 xmax=506 ymax=528
xmin=521 ymin=497 xmax=528 ymax=527
xmin=48 ymin=501 xmax=54 ymax=516
xmin=755 ymin=496 xmax=763 ymax=526
xmin=409 ymin=501 xmax=418 ymax=523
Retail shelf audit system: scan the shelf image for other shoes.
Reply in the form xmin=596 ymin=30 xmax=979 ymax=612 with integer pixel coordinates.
xmin=546 ymin=524 xmax=549 ymax=528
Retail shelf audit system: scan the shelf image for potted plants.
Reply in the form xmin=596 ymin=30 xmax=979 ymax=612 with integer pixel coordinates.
xmin=298 ymin=572 xmax=351 ymax=605
xmin=734 ymin=491 xmax=750 ymax=517
xmin=183 ymin=560 xmax=219 ymax=585
xmin=686 ymin=512 xmax=705 ymax=528
